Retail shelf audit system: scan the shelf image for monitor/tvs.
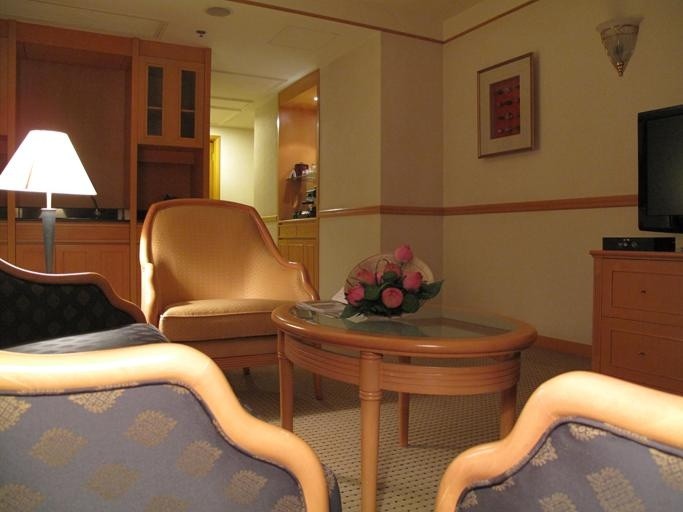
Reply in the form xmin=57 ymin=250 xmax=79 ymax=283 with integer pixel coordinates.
xmin=636 ymin=103 xmax=683 ymax=234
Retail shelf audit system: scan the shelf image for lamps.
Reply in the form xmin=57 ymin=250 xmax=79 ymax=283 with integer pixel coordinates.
xmin=596 ymin=17 xmax=644 ymax=76
xmin=0 ymin=129 xmax=98 ymax=275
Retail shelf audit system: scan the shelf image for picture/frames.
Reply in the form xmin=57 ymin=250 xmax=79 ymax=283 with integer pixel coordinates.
xmin=476 ymin=51 xmax=535 ymax=159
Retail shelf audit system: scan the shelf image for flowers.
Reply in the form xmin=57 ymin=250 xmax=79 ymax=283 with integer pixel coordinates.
xmin=339 ymin=242 xmax=446 ymax=319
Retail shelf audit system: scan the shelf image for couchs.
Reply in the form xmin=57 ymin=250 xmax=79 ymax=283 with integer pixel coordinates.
xmin=0 ymin=259 xmax=341 ymax=512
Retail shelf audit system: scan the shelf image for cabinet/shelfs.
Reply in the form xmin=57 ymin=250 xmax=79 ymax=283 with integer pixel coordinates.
xmin=136 ymin=39 xmax=210 ymax=151
xmin=12 ymin=220 xmax=132 ymax=307
xmin=276 ymin=218 xmax=317 ymax=290
xmin=589 ymin=250 xmax=683 ymax=397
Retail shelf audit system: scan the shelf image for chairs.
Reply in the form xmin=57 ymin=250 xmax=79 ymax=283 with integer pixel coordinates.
xmin=432 ymin=371 xmax=683 ymax=512
xmin=138 ymin=197 xmax=323 ymax=402
